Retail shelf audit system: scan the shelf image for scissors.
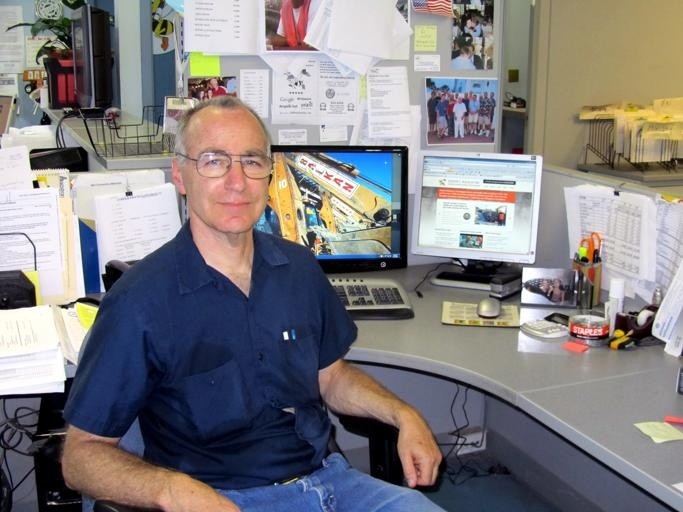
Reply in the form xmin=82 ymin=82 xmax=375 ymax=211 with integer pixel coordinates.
xmin=579 ymin=232 xmax=601 ymax=264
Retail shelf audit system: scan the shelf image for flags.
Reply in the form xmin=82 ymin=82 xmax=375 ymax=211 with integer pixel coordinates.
xmin=411 ymin=0 xmax=455 ymax=17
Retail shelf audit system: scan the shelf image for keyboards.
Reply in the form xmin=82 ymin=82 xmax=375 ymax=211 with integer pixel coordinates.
xmin=328 ymin=278 xmax=414 ymax=319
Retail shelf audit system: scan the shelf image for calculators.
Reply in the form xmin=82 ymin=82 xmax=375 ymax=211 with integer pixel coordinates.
xmin=518 ymin=313 xmax=569 ymax=338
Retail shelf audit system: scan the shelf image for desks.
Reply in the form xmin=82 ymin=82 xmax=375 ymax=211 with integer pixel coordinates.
xmin=27 ymin=86 xmax=175 ymax=172
xmin=321 ymin=262 xmax=683 ymax=510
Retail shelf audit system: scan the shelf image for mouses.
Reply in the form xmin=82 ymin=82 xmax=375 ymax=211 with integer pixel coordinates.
xmin=478 ymin=298 xmax=501 ymax=317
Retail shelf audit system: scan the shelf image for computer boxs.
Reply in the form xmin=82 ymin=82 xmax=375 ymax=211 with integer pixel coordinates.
xmin=33 ymin=396 xmax=82 ymax=512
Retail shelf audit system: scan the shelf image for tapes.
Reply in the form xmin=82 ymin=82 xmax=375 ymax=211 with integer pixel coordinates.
xmin=636 ymin=309 xmax=654 ymax=327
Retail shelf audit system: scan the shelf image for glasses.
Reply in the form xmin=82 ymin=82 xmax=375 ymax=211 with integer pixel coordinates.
xmin=176 ymin=150 xmax=274 ymax=180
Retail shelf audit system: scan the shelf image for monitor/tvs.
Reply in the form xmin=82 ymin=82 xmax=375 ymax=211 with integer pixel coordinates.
xmin=71 ymin=4 xmax=112 ymax=108
xmin=411 ymin=151 xmax=543 ymax=291
xmin=254 ymin=145 xmax=408 ymax=273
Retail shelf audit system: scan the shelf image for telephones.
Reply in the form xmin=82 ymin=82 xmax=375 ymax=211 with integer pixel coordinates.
xmin=101 ymin=260 xmax=142 ymax=294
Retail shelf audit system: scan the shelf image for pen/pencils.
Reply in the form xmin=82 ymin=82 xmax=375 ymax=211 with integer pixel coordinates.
xmin=572 ymin=247 xmax=602 ymax=307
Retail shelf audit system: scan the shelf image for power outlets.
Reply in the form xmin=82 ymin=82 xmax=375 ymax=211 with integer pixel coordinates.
xmin=454 ymin=429 xmax=489 ymax=457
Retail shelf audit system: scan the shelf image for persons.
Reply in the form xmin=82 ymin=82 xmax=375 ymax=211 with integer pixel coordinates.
xmin=451 ymin=0 xmax=493 ymax=73
xmin=188 ymin=76 xmax=236 ymax=101
xmin=60 ymin=95 xmax=447 ymax=512
xmin=427 ymin=85 xmax=496 ymax=138
